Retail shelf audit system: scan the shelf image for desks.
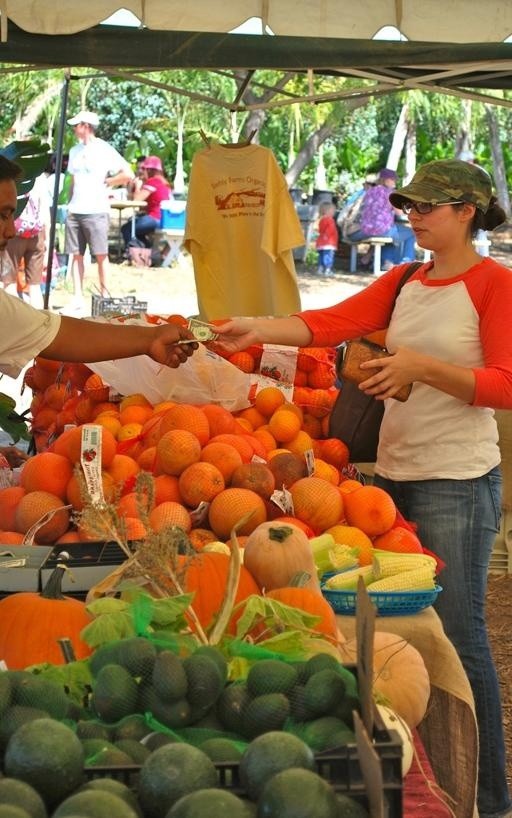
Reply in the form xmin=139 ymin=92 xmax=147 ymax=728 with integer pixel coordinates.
xmin=110 ymin=199 xmax=145 ymax=265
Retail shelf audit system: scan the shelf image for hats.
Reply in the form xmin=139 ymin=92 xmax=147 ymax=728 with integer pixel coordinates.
xmin=365 ymin=174 xmax=378 ymax=182
xmin=380 ymin=169 xmax=399 ymax=181
xmin=140 ymin=156 xmax=164 ymax=172
xmin=66 ymin=110 xmax=100 ymax=128
xmin=388 ymin=157 xmax=493 ymax=215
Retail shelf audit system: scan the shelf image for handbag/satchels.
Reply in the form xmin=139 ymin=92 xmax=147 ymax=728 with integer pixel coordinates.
xmin=128 ymin=246 xmax=153 ymax=268
xmin=325 ymin=263 xmax=427 ymax=462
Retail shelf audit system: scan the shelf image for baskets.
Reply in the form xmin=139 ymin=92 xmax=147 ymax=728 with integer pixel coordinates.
xmin=78 ymin=659 xmax=406 ymax=817
xmin=321 ymin=572 xmax=443 ymax=618
xmin=91 ymin=294 xmax=148 ymax=318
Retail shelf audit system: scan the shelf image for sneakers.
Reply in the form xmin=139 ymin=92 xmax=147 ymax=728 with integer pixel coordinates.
xmin=324 ymin=270 xmax=334 ymax=276
xmin=316 ymin=270 xmax=324 ymax=275
xmin=358 ymin=255 xmax=421 ymax=273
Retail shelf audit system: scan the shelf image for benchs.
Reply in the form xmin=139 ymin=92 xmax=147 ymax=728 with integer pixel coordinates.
xmin=151 ymin=228 xmax=188 ymax=267
xmin=339 ymin=236 xmax=387 ymax=275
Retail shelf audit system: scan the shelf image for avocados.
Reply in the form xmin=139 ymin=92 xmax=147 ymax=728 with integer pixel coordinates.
xmin=1 ymin=637 xmax=358 ymax=767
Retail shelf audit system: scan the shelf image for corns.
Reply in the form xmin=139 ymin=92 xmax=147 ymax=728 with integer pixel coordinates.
xmin=312 ymin=528 xmax=436 ymax=592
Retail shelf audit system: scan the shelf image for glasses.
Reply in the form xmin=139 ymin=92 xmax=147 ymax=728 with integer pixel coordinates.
xmin=401 ymin=201 xmax=460 ymax=215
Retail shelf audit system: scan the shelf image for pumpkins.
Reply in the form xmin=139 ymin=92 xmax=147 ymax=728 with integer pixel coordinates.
xmin=263 ymin=578 xmax=339 ymax=645
xmin=137 ymin=526 xmax=258 ymax=639
xmin=344 ymin=632 xmax=430 ymax=730
xmin=0 ymin=567 xmax=94 ymax=667
xmin=243 ymin=521 xmax=310 ymax=586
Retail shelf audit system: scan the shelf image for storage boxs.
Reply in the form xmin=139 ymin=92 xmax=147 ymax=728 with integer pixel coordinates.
xmin=159 ymin=199 xmax=189 ymax=229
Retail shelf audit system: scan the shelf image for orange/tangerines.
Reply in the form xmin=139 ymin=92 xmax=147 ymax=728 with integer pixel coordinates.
xmin=0 ymin=716 xmax=373 ymax=818
xmin=1 ymin=313 xmax=424 ymax=552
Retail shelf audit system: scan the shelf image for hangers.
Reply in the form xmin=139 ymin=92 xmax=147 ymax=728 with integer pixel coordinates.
xmin=213 ymin=110 xmax=247 ymax=144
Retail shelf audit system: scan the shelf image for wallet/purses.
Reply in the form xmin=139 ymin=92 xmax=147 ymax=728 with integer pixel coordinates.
xmin=335 ymin=337 xmax=415 ymax=402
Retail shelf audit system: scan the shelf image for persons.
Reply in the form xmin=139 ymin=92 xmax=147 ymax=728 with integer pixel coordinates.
xmin=209 ymin=160 xmax=512 ymax=818
xmin=316 ymin=167 xmax=415 ymax=277
xmin=0 ymin=154 xmax=199 ymax=380
xmin=6 ymin=110 xmax=176 ymax=312
